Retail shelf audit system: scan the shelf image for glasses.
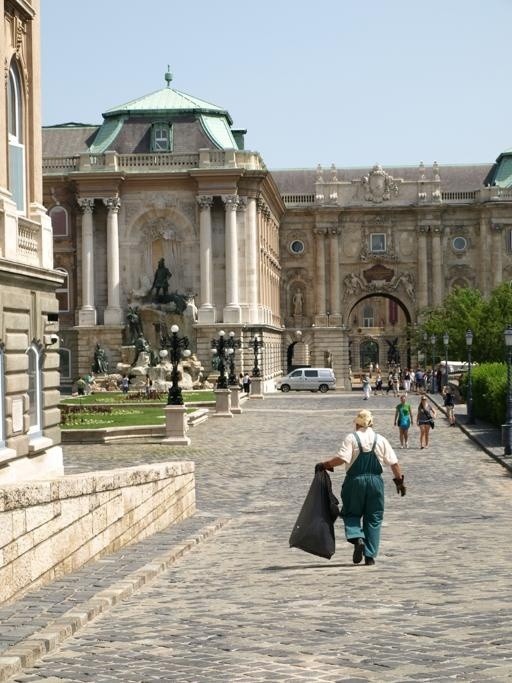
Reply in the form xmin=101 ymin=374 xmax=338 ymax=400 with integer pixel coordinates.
xmin=422 ymin=399 xmax=426 ymax=400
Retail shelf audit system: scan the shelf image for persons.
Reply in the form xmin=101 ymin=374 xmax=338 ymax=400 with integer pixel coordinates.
xmin=394 ymin=396 xmax=436 ymax=449
xmin=393 ymin=271 xmax=415 ymax=303
xmin=445 ymin=393 xmax=455 ymax=427
xmin=344 ymin=271 xmax=365 ymax=303
xmin=145 ymin=374 xmax=150 ymax=393
xmin=293 ymin=287 xmax=305 ymax=315
xmin=148 ymin=258 xmax=172 ymax=296
xmin=361 ymin=364 xmax=445 ymax=400
xmin=76 ymin=376 xmax=86 ymax=395
xmin=122 ymin=374 xmax=129 ymax=393
xmin=315 ymin=409 xmax=407 ymax=565
xmin=94 ymin=343 xmax=107 ymax=373
xmin=239 ymin=373 xmax=250 ymax=392
xmin=127 ymin=304 xmax=145 ymax=368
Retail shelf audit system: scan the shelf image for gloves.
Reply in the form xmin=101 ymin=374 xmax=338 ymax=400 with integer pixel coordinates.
xmin=315 ymin=462 xmax=332 ymax=471
xmin=392 ymin=475 xmax=405 ymax=496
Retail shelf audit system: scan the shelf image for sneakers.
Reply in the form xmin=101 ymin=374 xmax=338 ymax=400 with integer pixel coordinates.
xmin=353 ymin=409 xmax=373 ymax=427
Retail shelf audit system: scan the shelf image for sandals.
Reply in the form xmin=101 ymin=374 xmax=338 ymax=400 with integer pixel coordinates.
xmin=400 ymin=446 xmax=409 ymax=449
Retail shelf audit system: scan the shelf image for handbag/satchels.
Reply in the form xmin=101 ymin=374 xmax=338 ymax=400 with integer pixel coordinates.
xmin=401 ymin=417 xmax=408 ymax=429
xmin=369 ymin=375 xmax=410 ymax=391
xmin=431 ymin=422 xmax=434 ymax=429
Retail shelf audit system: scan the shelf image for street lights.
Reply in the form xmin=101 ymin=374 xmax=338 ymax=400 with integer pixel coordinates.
xmin=423 ymin=332 xmax=449 ymax=394
xmin=465 ymin=328 xmax=473 ymax=425
xmin=160 ymin=324 xmax=191 ymax=445
xmin=210 ymin=330 xmax=263 ymax=418
xmin=503 ymin=325 xmax=512 ymax=455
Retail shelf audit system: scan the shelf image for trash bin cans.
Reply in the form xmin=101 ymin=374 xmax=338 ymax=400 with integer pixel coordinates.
xmin=501 ymin=424 xmax=512 ymax=456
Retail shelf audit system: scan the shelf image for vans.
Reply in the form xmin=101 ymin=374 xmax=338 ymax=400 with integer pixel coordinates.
xmin=277 ymin=368 xmax=336 ymax=393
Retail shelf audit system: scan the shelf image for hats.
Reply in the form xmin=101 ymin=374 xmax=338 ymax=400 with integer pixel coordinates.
xmin=353 ymin=538 xmax=375 ymax=565
